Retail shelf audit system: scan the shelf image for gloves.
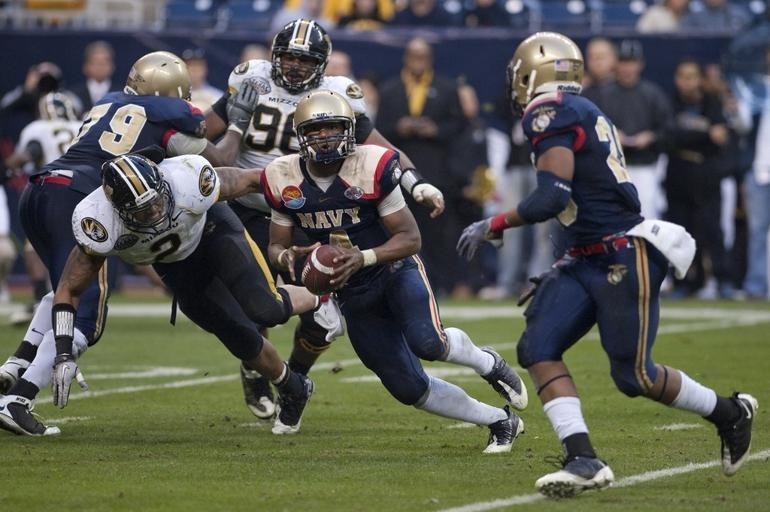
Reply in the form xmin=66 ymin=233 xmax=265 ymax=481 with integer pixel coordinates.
xmin=51 ymin=353 xmax=88 ymax=409
xmin=412 ymin=182 xmax=444 ymax=208
xmin=518 ymin=269 xmax=553 ymax=316
xmin=456 ymin=217 xmax=504 ymax=263
xmin=226 ymin=82 xmax=260 ymax=134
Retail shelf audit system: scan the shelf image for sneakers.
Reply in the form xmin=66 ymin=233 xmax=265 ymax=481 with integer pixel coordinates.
xmin=0 ymin=361 xmax=36 ymax=411
xmin=717 ymin=391 xmax=759 ymax=477
xmin=478 ymin=345 xmax=528 ymax=411
xmin=0 ymin=395 xmax=60 ymax=436
xmin=482 ymin=404 xmax=525 ymax=453
xmin=240 ymin=361 xmax=276 ymax=418
xmin=271 ymin=372 xmax=314 ymax=434
xmin=535 ymin=451 xmax=615 ymax=500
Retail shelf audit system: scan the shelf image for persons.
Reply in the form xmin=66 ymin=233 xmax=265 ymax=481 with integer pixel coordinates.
xmin=376 ymin=37 xmax=465 ymax=299
xmin=0 ymin=54 xmax=66 ymax=276
xmin=476 ymin=91 xmax=555 ymax=303
xmin=183 ymin=47 xmax=223 ymax=115
xmin=456 ymin=32 xmax=759 ymax=498
xmin=0 ymin=51 xmax=258 ymax=437
xmin=9 ymin=87 xmax=81 ymax=178
xmin=261 ymin=89 xmax=529 ymax=454
xmin=201 ymin=19 xmax=445 ymax=437
xmin=67 ymin=40 xmax=123 ymax=110
xmin=581 ymin=39 xmax=770 ymax=304
xmin=51 ymin=154 xmax=346 ymax=411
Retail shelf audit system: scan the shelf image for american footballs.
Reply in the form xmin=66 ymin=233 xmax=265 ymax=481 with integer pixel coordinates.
xmin=301 ymin=244 xmax=344 ymax=296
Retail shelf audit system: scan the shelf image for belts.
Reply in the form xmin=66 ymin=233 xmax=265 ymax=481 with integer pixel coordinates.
xmin=566 ymin=236 xmax=626 ymax=258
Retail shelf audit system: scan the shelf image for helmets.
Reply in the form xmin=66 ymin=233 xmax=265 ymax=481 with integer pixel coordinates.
xmin=504 ymin=32 xmax=584 ymax=119
xmin=102 ymin=153 xmax=175 ymax=235
xmin=123 ymin=50 xmax=192 ymax=102
xmin=294 ymin=89 xmax=358 ymax=164
xmin=270 ymin=19 xmax=332 ymax=95
